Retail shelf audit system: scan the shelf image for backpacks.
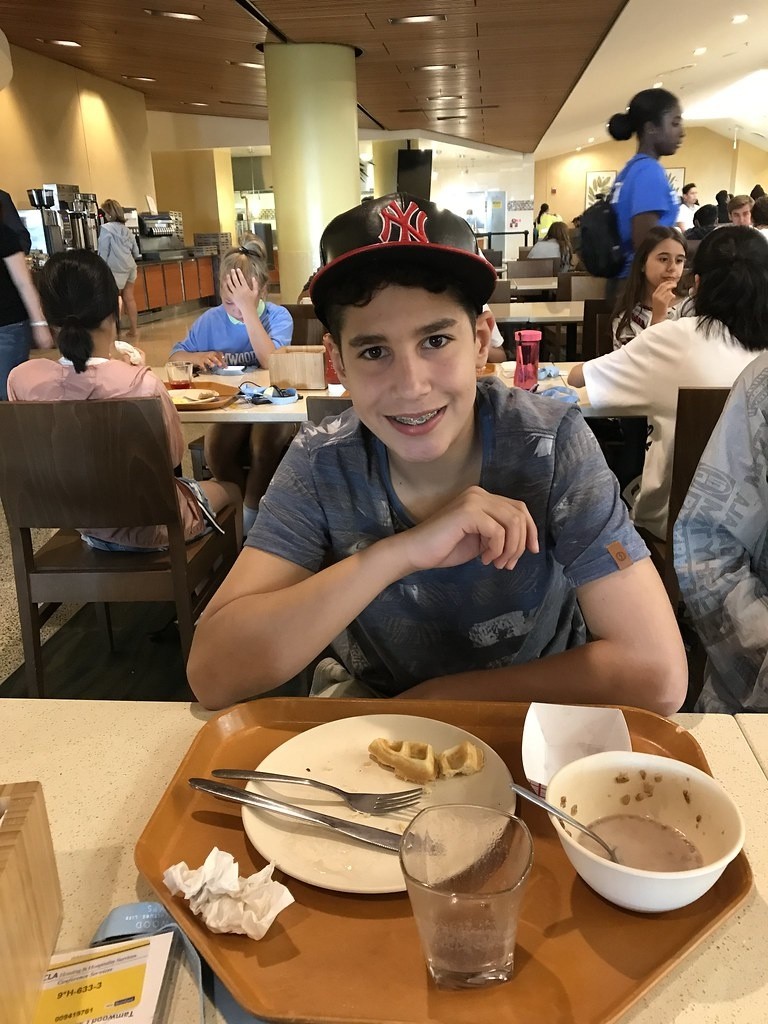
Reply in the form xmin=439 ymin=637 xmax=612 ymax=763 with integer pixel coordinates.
xmin=581 ymin=157 xmax=633 ymax=277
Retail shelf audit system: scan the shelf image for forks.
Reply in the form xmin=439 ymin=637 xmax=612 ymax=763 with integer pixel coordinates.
xmin=211 ymin=769 xmax=422 ymax=816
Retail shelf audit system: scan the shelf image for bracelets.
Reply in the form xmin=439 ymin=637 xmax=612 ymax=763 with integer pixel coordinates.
xmin=30 ymin=321 xmax=49 ymax=327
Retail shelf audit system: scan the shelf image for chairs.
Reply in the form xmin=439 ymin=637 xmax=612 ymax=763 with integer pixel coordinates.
xmin=282 ymin=248 xmax=624 ymax=361
xmin=644 ymin=388 xmax=729 ymax=626
xmin=0 ymin=397 xmax=235 ymax=696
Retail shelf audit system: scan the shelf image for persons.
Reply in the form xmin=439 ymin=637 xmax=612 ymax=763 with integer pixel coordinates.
xmin=528 ymin=89 xmax=768 ymax=715
xmin=169 ymin=233 xmax=294 ymax=544
xmin=7 ymin=248 xmax=243 ymax=635
xmin=0 ymin=190 xmax=55 ymax=401
xmin=483 ymin=304 xmax=508 ymax=363
xmin=98 ymin=199 xmax=139 ymax=337
xmin=186 ymin=192 xmax=689 ymax=718
xmin=168 ymin=232 xmax=300 ymax=545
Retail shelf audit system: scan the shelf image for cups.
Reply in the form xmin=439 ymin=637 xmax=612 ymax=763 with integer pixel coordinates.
xmin=165 ymin=360 xmax=194 ymax=390
xmin=398 ymin=804 xmax=534 ymax=988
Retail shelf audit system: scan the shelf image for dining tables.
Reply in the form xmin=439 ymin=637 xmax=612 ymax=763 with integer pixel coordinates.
xmin=0 ymin=693 xmax=768 ymax=1024
xmin=173 ymin=361 xmax=624 ymax=422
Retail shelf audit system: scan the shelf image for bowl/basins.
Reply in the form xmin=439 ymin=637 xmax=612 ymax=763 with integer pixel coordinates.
xmin=545 ymin=751 xmax=745 ymax=911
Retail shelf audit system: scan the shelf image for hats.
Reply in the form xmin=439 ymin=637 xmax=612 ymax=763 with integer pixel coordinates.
xmin=309 ymin=193 xmax=497 ymax=331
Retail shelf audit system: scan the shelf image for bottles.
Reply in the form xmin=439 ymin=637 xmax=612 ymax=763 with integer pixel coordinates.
xmin=514 ymin=329 xmax=542 ymax=391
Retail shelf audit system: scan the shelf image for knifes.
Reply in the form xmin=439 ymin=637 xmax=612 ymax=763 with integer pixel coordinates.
xmin=185 ymin=775 xmax=424 ymax=856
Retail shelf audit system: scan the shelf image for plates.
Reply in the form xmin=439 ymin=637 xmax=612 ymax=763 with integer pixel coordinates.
xmin=164 ymin=389 xmax=219 ymax=402
xmin=242 ymin=714 xmax=516 ymax=894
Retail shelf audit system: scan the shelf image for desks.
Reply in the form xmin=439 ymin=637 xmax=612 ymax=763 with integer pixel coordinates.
xmin=484 ymin=300 xmax=586 ymax=356
xmin=495 ymin=273 xmax=559 ymax=300
